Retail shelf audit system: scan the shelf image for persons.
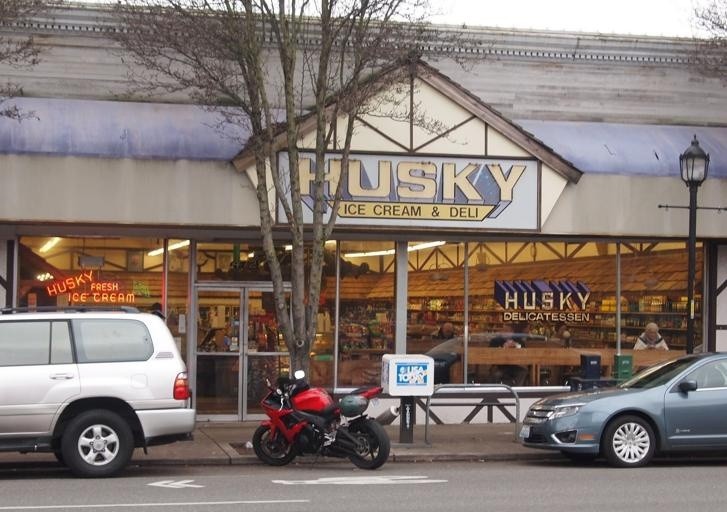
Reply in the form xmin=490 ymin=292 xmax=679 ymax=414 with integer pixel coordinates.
xmin=489 ymin=323 xmax=528 ymax=386
xmin=549 ymin=322 xmax=574 ymax=345
xmin=633 ymin=322 xmax=671 ymax=350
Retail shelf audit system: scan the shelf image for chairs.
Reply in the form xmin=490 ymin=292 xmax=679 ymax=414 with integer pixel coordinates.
xmin=696 ymin=367 xmax=724 ymax=387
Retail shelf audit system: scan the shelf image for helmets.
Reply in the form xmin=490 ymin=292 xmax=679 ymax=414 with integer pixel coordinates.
xmin=340 ymin=395 xmax=368 ymax=418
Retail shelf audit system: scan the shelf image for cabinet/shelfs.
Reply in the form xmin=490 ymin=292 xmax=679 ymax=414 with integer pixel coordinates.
xmin=139 ymin=288 xmax=702 ymax=350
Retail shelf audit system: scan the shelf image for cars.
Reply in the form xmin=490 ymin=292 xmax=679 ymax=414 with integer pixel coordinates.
xmin=424 ymin=332 xmax=559 ymax=386
xmin=518 ymin=352 xmax=726 ymax=468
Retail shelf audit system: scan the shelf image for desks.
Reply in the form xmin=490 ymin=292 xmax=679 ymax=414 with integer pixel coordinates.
xmin=455 ymin=346 xmax=687 ymax=387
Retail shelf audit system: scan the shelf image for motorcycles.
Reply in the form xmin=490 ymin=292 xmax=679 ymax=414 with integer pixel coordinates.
xmin=252 ymin=364 xmax=398 ymax=470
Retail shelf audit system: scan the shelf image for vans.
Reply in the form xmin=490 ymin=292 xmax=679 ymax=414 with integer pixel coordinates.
xmin=0 ymin=305 xmax=196 ymax=477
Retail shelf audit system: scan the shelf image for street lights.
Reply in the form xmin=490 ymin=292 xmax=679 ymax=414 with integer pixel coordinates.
xmin=678 ymin=134 xmax=710 ymax=353
